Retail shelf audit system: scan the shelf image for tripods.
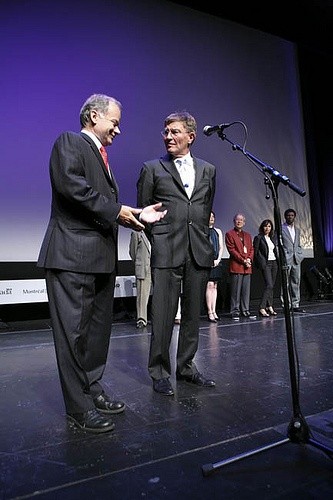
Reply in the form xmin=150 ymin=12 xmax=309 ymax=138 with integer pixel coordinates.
xmin=200 ymin=131 xmax=333 ymax=478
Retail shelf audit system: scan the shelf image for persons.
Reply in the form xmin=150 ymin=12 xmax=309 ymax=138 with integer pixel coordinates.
xmin=129 ymin=207 xmax=304 ymax=329
xmin=36 ymin=93 xmax=168 ymax=432
xmin=138 ymin=111 xmax=218 ymax=395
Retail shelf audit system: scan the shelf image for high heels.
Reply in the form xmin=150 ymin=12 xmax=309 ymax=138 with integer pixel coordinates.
xmin=267 ymin=308 xmax=278 ymax=316
xmin=258 ymin=309 xmax=269 ymax=317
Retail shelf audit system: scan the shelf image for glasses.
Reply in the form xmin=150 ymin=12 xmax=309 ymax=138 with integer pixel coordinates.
xmin=160 ymin=129 xmax=190 ymax=138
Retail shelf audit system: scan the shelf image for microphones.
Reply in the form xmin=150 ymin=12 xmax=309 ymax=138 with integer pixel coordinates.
xmin=203 ymin=123 xmax=231 ymax=136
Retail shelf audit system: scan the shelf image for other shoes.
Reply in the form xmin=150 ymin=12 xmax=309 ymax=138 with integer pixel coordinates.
xmin=215 ymin=317 xmax=221 ymax=321
xmin=136 ymin=322 xmax=144 ymax=328
xmin=242 ymin=311 xmax=251 ymax=318
xmin=208 ymin=314 xmax=217 ymax=323
xmin=292 ymin=308 xmax=307 ymax=314
xmin=174 ymin=319 xmax=181 ymax=324
xmin=231 ymin=314 xmax=240 ymax=320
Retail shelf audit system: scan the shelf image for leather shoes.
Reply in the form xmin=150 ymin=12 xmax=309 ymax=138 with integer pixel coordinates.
xmin=66 ymin=408 xmax=115 ymax=434
xmin=93 ymin=390 xmax=126 ymax=414
xmin=176 ymin=372 xmax=216 ymax=387
xmin=153 ymin=378 xmax=174 ymax=396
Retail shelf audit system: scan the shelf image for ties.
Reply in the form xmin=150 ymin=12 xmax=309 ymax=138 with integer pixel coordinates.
xmin=100 ymin=146 xmax=108 ymax=173
xmin=179 ymin=159 xmax=186 ymax=173
